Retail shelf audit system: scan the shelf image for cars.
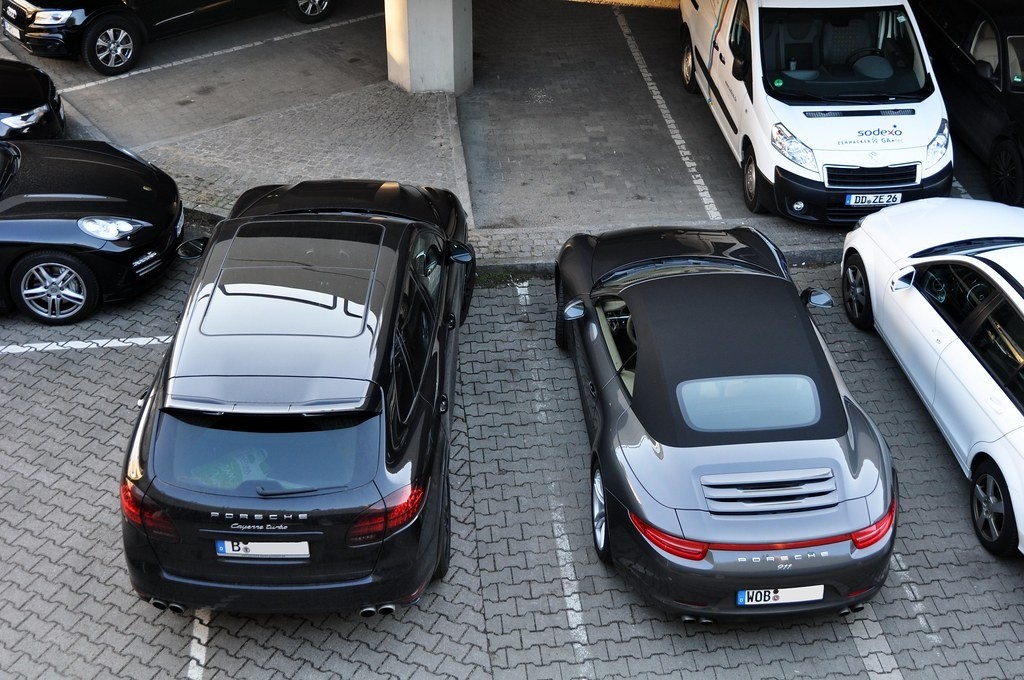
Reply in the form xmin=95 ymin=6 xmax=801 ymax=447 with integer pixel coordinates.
xmin=0 ymin=0 xmax=339 ymax=78
xmin=839 ymin=198 xmax=1024 ymax=562
xmin=0 ymin=140 xmax=184 ymax=324
xmin=913 ymin=1 xmax=1024 ymax=205
xmin=0 ymin=54 xmax=66 ymax=143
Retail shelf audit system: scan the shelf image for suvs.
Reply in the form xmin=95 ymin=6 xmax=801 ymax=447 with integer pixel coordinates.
xmin=118 ymin=177 xmax=477 ymax=620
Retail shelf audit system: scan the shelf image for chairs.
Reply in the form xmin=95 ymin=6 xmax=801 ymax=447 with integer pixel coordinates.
xmin=973 ymin=23 xmax=1021 ymax=83
xmin=765 ymin=19 xmax=821 ymax=67
xmin=823 ymin=18 xmax=873 ymax=66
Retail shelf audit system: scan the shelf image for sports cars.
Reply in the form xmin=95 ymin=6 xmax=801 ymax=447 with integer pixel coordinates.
xmin=552 ymin=224 xmax=900 ymax=630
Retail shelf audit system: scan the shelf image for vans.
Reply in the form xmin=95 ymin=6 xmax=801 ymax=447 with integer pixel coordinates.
xmin=680 ymin=0 xmax=956 ymax=228
